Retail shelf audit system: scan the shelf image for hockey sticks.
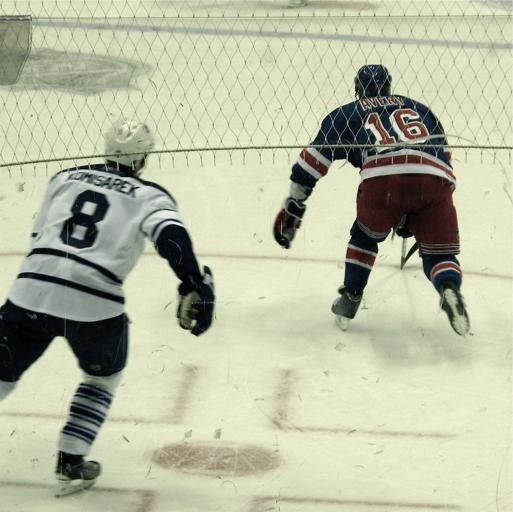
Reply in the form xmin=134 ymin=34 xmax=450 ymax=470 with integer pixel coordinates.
xmin=400 ymin=238 xmax=418 ymax=269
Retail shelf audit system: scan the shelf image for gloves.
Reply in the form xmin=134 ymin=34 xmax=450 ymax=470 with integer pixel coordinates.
xmin=273 ymin=198 xmax=307 ymax=250
xmin=175 ymin=266 xmax=214 ymax=336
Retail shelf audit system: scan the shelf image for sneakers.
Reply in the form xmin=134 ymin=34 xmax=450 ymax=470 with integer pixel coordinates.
xmin=55 ymin=451 xmax=101 ymax=481
xmin=331 ymin=286 xmax=364 ymax=320
xmin=438 ymin=280 xmax=471 ymax=336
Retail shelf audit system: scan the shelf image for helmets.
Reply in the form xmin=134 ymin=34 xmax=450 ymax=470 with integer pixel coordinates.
xmin=105 ymin=118 xmax=154 ymax=173
xmin=354 ymin=64 xmax=392 ymax=99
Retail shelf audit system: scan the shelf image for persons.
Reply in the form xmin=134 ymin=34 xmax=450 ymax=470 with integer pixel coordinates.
xmin=0 ymin=110 xmax=215 ymax=481
xmin=272 ymin=64 xmax=471 ymax=336
